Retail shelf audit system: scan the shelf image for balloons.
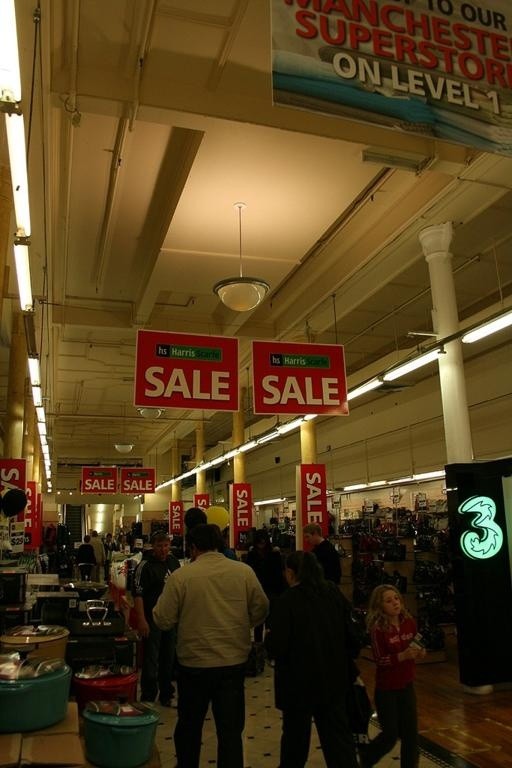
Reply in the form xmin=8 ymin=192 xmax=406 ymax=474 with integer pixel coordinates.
xmin=2 ymin=489 xmax=27 ymax=516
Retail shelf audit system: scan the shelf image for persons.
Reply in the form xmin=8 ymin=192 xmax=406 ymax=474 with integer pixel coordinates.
xmin=75 ymin=506 xmax=358 ymax=768
xmin=357 ymin=584 xmax=426 ymax=768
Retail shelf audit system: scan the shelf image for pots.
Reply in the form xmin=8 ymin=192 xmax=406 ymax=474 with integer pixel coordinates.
xmin=0 ymin=624 xmax=71 ymax=659
xmin=73 ymin=664 xmax=139 ymax=717
xmin=83 ymin=702 xmax=160 ymax=768
xmin=0 ymin=655 xmax=72 ymax=732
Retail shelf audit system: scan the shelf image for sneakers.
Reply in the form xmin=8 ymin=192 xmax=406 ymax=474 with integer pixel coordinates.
xmin=156 ymin=694 xmax=180 ymax=708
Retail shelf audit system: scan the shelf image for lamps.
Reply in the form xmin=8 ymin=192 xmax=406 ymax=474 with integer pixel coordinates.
xmin=0 ymin=0 xmax=53 ymax=494
xmin=113 ymin=401 xmax=136 ymax=455
xmin=462 ymin=311 xmax=512 ymax=346
xmin=213 ymin=203 xmax=270 ymax=313
